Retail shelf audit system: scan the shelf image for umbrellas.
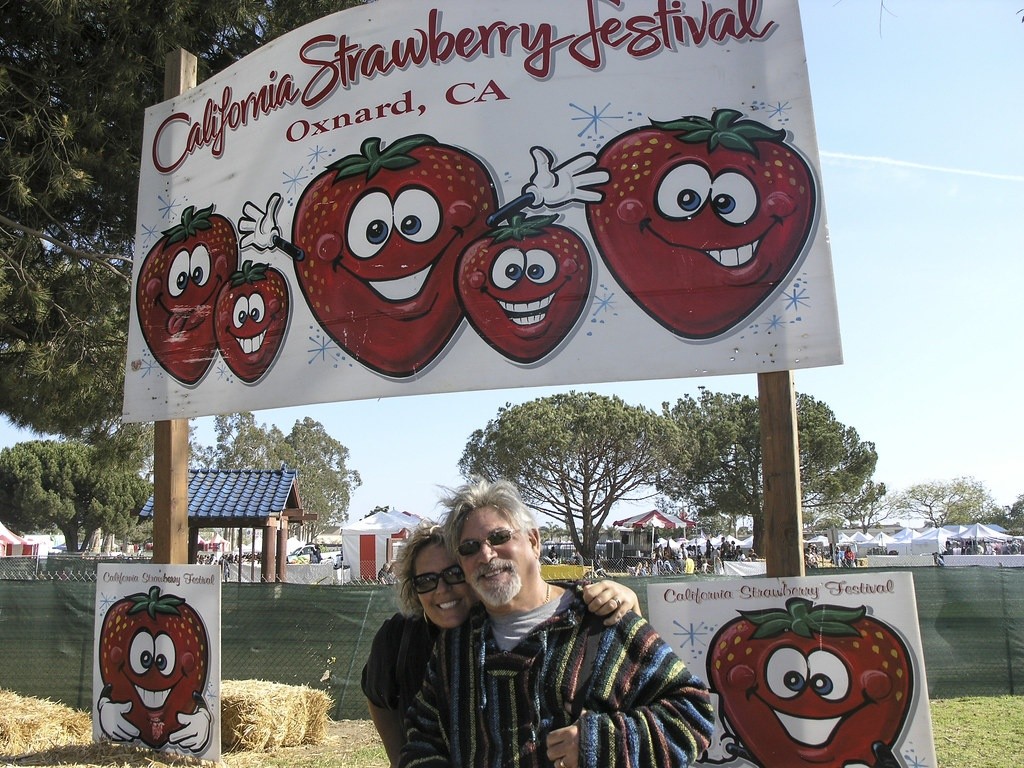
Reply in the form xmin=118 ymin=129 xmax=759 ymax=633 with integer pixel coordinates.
xmin=612 ymin=510 xmax=699 ymax=560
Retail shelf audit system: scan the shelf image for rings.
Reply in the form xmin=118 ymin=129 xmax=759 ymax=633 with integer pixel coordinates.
xmin=559 ymin=758 xmax=567 ymax=767
xmin=611 ymin=596 xmax=621 ymax=606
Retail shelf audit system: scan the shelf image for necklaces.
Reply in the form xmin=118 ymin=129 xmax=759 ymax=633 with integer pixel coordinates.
xmin=544 ymin=583 xmax=551 ymax=603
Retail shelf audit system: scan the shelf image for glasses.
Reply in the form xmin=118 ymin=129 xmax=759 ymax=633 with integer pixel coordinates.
xmin=410 ymin=564 xmax=466 ymax=594
xmin=456 ymin=527 xmax=521 ymax=557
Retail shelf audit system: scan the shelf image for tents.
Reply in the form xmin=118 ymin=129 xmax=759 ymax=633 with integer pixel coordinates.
xmin=804 ymin=523 xmax=1013 ymax=553
xmin=653 ymin=533 xmax=754 ymax=550
xmin=339 ymin=510 xmax=437 ymax=583
xmin=198 ymin=534 xmax=304 ymax=555
xmin=0 ymin=521 xmax=42 ymax=556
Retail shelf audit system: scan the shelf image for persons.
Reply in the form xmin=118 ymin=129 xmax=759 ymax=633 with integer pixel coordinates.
xmin=548 ymin=546 xmax=604 ymax=569
xmin=942 ymin=540 xmax=1023 ymax=555
xmin=397 ymin=478 xmax=715 ymax=768
xmin=804 ymin=542 xmax=858 ymax=572
xmin=361 ymin=523 xmax=642 ymax=768
xmin=199 ymin=552 xmax=263 ymax=577
xmin=632 ymin=537 xmax=758 ymax=576
